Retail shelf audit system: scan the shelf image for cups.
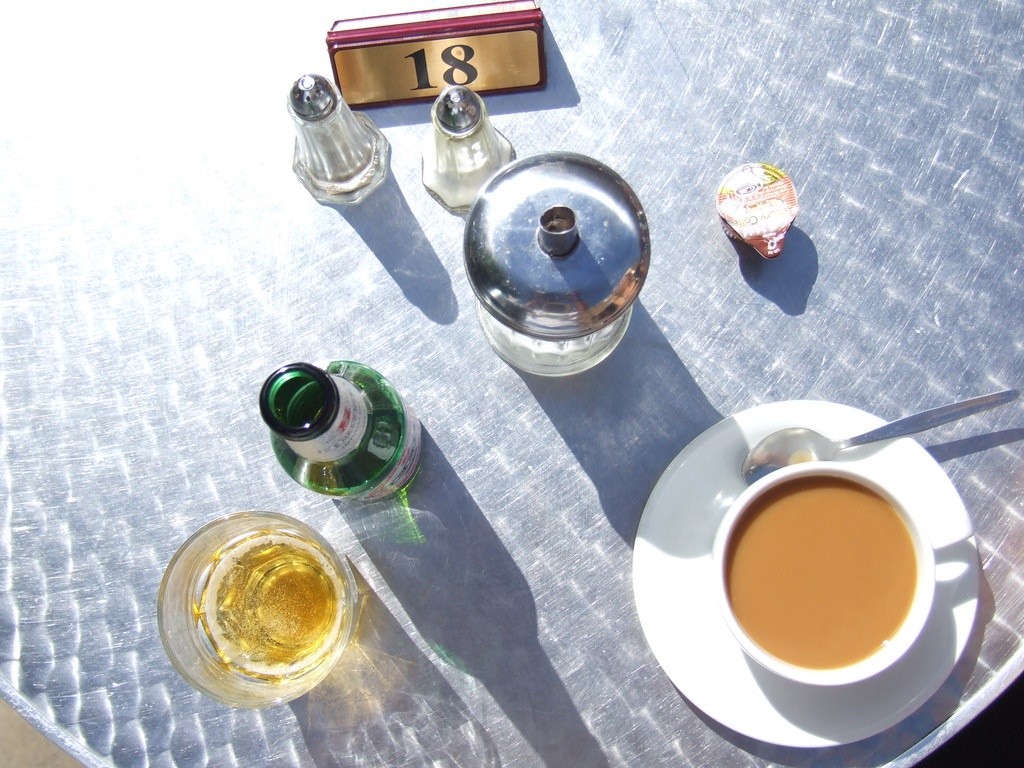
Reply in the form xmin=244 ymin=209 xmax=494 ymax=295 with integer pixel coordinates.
xmin=157 ymin=511 xmax=353 ymax=708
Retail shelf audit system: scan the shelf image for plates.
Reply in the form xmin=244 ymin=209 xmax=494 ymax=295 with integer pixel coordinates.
xmin=631 ymin=400 xmax=983 ymax=746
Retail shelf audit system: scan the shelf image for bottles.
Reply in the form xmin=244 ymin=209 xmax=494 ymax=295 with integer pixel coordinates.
xmin=421 ymin=86 xmax=517 ymax=212
xmin=287 ymin=74 xmax=390 ymax=206
xmin=261 ymin=362 xmax=423 ymax=503
xmin=463 ymin=152 xmax=652 ymax=375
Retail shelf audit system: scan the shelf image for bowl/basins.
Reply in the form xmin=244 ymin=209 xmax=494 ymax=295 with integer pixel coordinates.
xmin=711 ymin=463 xmax=933 ymax=684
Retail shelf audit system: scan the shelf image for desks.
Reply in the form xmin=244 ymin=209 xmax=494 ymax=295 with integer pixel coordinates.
xmin=0 ymin=0 xmax=1024 ymax=768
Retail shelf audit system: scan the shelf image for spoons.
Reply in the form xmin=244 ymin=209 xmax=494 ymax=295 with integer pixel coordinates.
xmin=743 ymin=389 xmax=1021 ymax=487
